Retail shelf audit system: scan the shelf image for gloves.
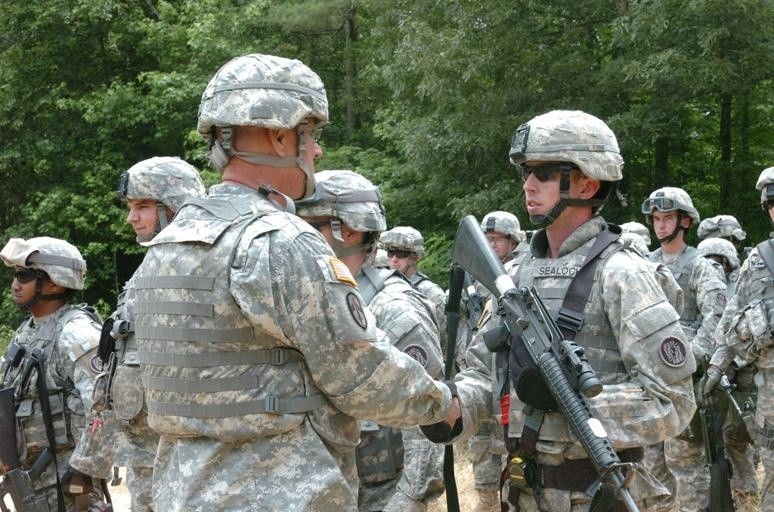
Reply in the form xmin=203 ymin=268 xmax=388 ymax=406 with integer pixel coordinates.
xmin=419 ymin=380 xmax=464 ymax=444
xmin=61 ymin=466 xmax=94 ymax=496
xmin=508 ymin=336 xmax=576 ymax=412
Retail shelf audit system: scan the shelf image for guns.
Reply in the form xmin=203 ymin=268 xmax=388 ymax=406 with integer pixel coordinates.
xmin=451 ymin=215 xmax=639 ymax=512
xmin=699 ymin=353 xmax=736 ymax=512
xmin=719 ymin=374 xmax=745 ymax=417
xmin=0 ymin=386 xmax=54 ymax=512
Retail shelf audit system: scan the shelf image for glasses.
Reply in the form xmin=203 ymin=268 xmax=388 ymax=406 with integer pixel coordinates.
xmin=641 ymin=197 xmax=679 ymax=214
xmin=766 ymin=200 xmax=774 ymax=207
xmin=14 ymin=269 xmax=36 ymax=284
xmin=520 ymin=164 xmax=579 ymax=182
xmin=388 ymin=250 xmax=416 ymax=258
xmin=308 ymin=221 xmax=330 ymax=231
xmin=765 ymin=184 xmax=774 ymax=196
xmin=487 ymin=235 xmax=506 ymax=243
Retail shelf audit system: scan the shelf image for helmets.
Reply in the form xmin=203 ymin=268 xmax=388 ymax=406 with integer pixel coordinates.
xmin=756 ymin=166 xmax=774 ymax=210
xmin=197 ymin=54 xmax=329 ymax=136
xmin=645 ymin=186 xmax=700 ymax=225
xmin=697 ymin=215 xmax=746 ymax=240
xmin=697 ymin=237 xmax=739 ymax=267
xmin=11 ymin=237 xmax=86 ymax=291
xmin=118 ymin=156 xmax=206 ymax=213
xmin=620 ymin=221 xmax=651 ymax=245
xmin=379 ymin=225 xmax=424 ymax=254
xmin=297 ymin=169 xmax=387 ymax=232
xmin=480 ymin=211 xmax=521 ymax=242
xmin=621 ymin=233 xmax=649 ymax=254
xmin=508 ymin=109 xmax=624 ymax=182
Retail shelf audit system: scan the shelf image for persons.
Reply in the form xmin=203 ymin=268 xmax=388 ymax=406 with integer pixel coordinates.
xmin=97 ymin=154 xmax=205 ymax=512
xmin=134 ymin=54 xmax=459 ymax=512
xmin=697 ymin=238 xmax=760 ymax=512
xmin=0 ymin=236 xmax=113 ymax=512
xmin=297 ymin=168 xmax=448 ymax=512
xmin=419 ymin=108 xmax=695 ymax=512
xmin=443 ymin=211 xmax=525 ymax=512
xmin=697 ymin=215 xmax=753 ymax=265
xmin=380 ymin=226 xmax=449 ymax=353
xmin=697 ymin=167 xmax=774 ymax=512
xmin=640 ymin=186 xmax=729 ymax=512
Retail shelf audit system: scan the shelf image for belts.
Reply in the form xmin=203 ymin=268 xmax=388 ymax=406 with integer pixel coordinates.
xmin=537 ymin=447 xmax=645 ymax=490
